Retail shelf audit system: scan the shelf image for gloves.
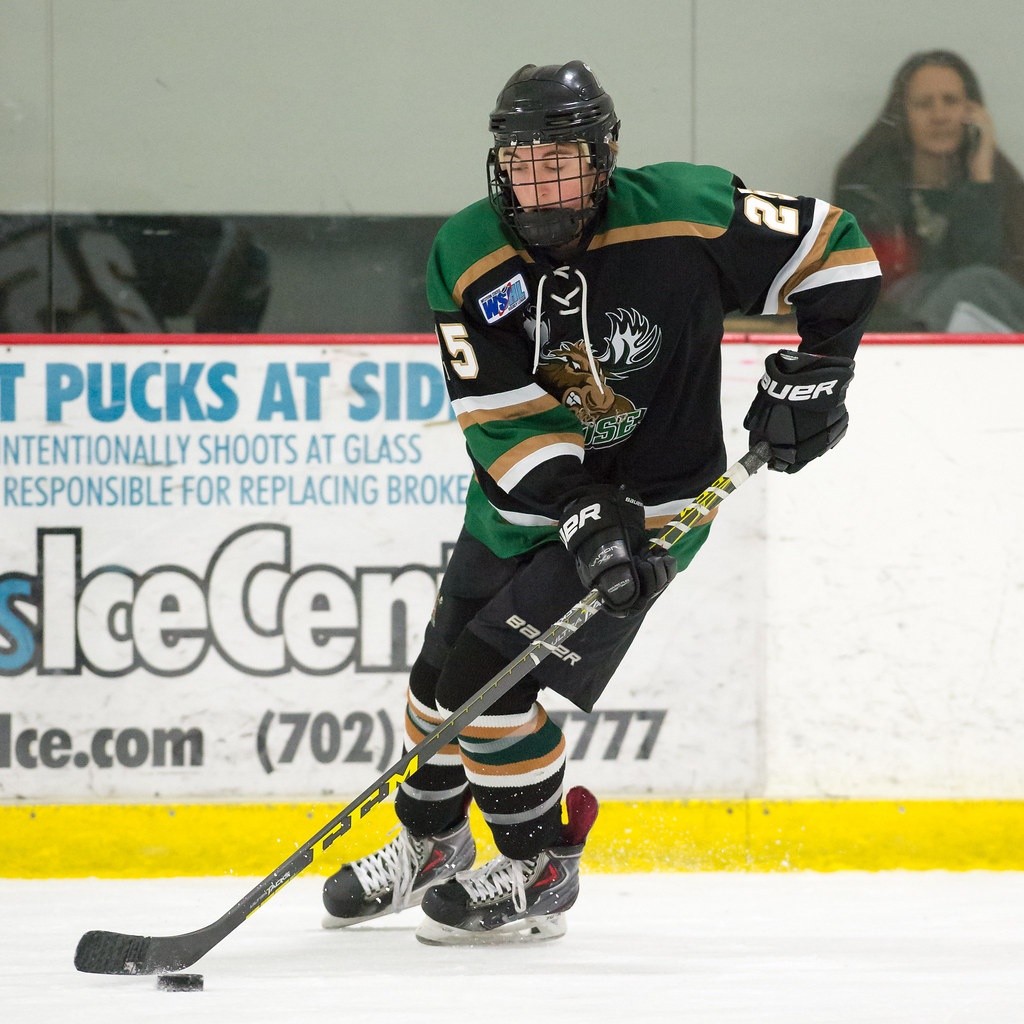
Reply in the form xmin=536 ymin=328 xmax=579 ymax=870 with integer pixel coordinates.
xmin=744 ymin=349 xmax=855 ymax=474
xmin=557 ymin=481 xmax=677 ymax=619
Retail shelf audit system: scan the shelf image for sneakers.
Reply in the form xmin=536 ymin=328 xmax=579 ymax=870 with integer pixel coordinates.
xmin=415 ymin=785 xmax=599 ymax=948
xmin=321 ymin=817 xmax=476 ymax=929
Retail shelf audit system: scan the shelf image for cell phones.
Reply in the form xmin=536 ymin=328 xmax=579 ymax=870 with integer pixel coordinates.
xmin=965 ymin=124 xmax=980 ymax=149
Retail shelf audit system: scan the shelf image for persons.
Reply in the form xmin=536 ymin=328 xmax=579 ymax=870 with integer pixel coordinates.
xmin=831 ymin=51 xmax=1022 ymax=333
xmin=320 ymin=63 xmax=885 ymax=934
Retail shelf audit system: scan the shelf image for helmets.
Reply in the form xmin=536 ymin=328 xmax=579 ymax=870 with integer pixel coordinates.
xmin=486 ymin=60 xmax=621 ymax=247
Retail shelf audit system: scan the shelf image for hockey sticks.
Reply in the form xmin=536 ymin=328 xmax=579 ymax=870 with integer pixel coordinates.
xmin=67 ymin=433 xmax=775 ymax=976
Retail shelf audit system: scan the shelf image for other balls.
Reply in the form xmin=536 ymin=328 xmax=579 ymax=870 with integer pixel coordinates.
xmin=156 ymin=975 xmax=205 ymax=994
